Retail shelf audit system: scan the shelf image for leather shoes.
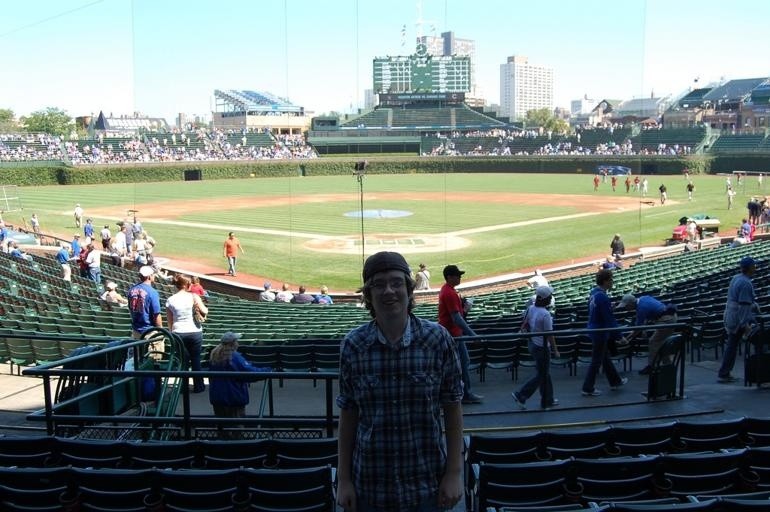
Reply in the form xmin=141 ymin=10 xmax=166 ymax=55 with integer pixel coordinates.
xmin=639 ymin=365 xmax=651 ymax=375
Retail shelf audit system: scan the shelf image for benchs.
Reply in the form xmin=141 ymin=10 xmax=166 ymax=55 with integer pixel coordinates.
xmin=2 ymin=128 xmax=280 ymax=158
xmin=418 ymin=124 xmax=770 ymax=159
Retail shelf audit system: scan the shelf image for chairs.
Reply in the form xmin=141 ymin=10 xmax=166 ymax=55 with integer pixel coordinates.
xmin=601 ymin=239 xmax=770 ymax=374
xmin=1 ymin=250 xmax=172 ymax=312
xmin=1 ymin=415 xmax=770 ymax=512
xmin=1 ymin=301 xmax=578 ymax=389
xmin=466 ymin=273 xmax=638 ymax=377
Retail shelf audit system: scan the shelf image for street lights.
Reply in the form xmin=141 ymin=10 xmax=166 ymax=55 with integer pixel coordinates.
xmin=352 ymin=160 xmax=369 ymax=282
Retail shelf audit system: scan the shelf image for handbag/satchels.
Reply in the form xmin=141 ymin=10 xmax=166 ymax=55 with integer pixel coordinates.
xmin=192 ymin=294 xmax=207 ymax=324
xmin=517 ymin=306 xmax=529 ymax=336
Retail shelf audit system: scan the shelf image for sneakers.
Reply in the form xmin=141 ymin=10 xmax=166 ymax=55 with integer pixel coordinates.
xmin=511 ymin=390 xmax=528 ymax=410
xmin=461 ymin=392 xmax=486 ymax=404
xmin=717 ymin=372 xmax=736 ymax=382
xmin=609 ymin=377 xmax=628 ymax=392
xmin=581 ymin=387 xmax=603 ymax=396
xmin=542 ymin=397 xmax=560 ymax=410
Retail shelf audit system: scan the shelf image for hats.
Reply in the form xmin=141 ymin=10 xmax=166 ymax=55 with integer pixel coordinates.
xmin=741 ymin=258 xmax=760 ymax=265
xmin=537 ymin=284 xmax=555 ymax=301
xmin=107 ymin=281 xmax=117 ymax=290
xmin=616 ymin=294 xmax=636 ymax=308
xmin=362 ymin=252 xmax=411 ymax=282
xmin=139 ymin=265 xmax=154 ymax=277
xmin=221 ymin=331 xmax=243 ymax=345
xmin=444 ymin=265 xmax=465 ymax=276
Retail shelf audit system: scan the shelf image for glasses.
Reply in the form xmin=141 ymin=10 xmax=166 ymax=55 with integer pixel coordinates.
xmin=368 ymin=280 xmax=407 ymax=289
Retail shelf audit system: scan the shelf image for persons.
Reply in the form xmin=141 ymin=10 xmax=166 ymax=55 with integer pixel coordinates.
xmin=716 ymin=256 xmax=760 ymax=383
xmin=438 ymin=264 xmax=484 ymax=405
xmin=615 ymin=294 xmax=677 ymax=375
xmin=335 ymin=252 xmax=465 ymax=512
xmin=511 ymin=285 xmax=560 ymax=410
xmin=580 ymin=269 xmax=629 ymax=396
